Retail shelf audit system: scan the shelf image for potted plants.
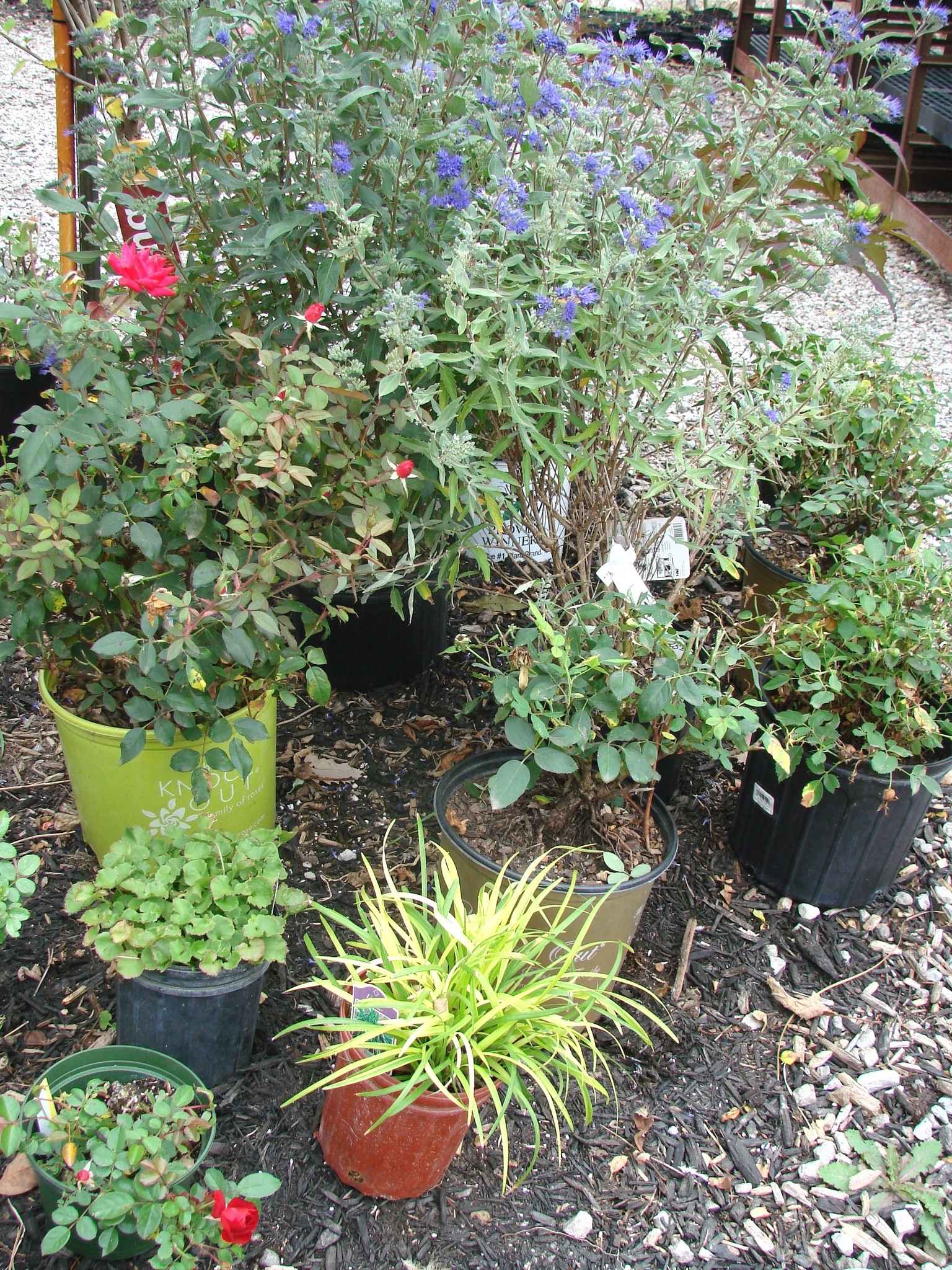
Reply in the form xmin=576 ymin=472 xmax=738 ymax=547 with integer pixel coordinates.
xmin=433 ymin=325 xmax=952 ymax=1034
xmin=274 ymin=805 xmax=679 ymax=1202
xmin=62 ymin=812 xmax=313 ymax=1087
xmin=0 ymin=219 xmax=78 ymax=475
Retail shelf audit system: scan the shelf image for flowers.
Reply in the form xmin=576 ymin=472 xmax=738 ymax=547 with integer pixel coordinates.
xmin=0 ymin=0 xmax=952 ymax=802
xmin=0 ymin=1075 xmax=282 ymax=1270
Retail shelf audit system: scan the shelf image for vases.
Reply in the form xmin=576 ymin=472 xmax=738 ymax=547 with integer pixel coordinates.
xmin=37 ymin=612 xmax=278 ymax=864
xmin=283 ymin=537 xmax=457 ymax=690
xmin=22 ymin=1045 xmax=217 ymax=1262
